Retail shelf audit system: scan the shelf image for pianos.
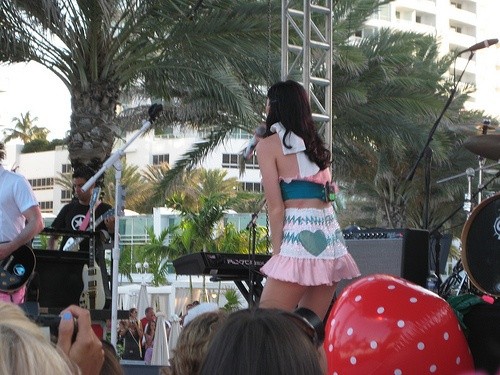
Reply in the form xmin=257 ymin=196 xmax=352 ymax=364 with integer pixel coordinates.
xmin=172 ymin=254 xmax=272 ymax=310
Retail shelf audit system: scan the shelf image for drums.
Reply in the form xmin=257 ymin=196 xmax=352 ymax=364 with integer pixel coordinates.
xmin=461 ymin=193 xmax=500 ymax=299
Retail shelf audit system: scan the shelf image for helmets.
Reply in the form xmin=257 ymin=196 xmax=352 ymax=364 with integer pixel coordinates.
xmin=324 ymin=274 xmax=475 ymax=375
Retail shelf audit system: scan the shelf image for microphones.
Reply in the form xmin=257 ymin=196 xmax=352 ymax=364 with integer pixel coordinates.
xmin=463 ymin=39 xmax=498 ymax=55
xmin=89 ymin=187 xmax=102 ymax=209
xmin=243 ymin=125 xmax=266 ymax=160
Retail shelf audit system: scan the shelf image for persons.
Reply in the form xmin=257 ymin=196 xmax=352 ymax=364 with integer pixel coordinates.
xmin=0 ymin=142 xmax=45 ymax=306
xmin=46 ymin=167 xmax=115 ymax=272
xmin=0 ymin=275 xmax=500 ymax=375
xmin=252 ymin=80 xmax=361 ymax=320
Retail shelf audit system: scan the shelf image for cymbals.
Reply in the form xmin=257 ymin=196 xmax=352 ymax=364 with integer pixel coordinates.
xmin=466 ymin=134 xmax=500 ymax=161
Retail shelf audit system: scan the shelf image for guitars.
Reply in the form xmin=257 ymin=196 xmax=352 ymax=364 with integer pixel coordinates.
xmin=0 ymin=240 xmax=36 ymax=293
xmin=79 ymin=186 xmax=106 ymax=310
xmin=62 ymin=207 xmax=115 ymax=251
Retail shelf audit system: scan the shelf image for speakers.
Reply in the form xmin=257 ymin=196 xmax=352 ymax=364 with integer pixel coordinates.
xmin=335 ymin=229 xmax=429 ymax=297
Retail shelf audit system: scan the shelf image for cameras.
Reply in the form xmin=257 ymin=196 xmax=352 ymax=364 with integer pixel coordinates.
xmin=32 ymin=314 xmax=79 ymax=345
xmin=130 ymin=320 xmax=135 ymax=325
